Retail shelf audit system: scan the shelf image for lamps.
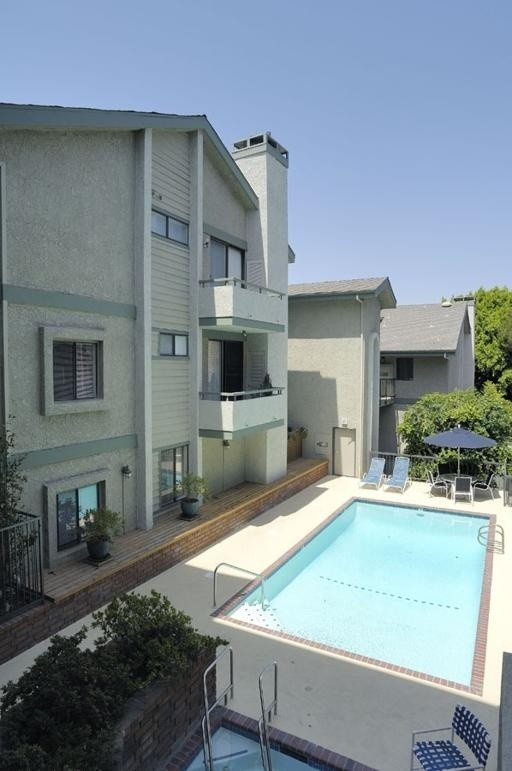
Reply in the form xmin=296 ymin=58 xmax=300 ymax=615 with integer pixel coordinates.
xmin=122 ymin=465 xmax=132 ymax=479
xmin=224 ymin=440 xmax=231 ymax=450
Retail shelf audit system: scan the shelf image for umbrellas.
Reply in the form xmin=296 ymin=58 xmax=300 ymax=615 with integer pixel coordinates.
xmin=423 ymin=424 xmax=498 ymax=476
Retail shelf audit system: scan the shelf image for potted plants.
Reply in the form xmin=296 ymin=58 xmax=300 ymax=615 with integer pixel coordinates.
xmin=76 ymin=506 xmax=126 ymax=567
xmin=172 ymin=474 xmax=209 ymax=522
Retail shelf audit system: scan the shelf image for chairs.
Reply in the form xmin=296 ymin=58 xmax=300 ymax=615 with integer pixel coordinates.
xmin=362 ymin=456 xmax=495 ymax=503
xmin=410 ymin=704 xmax=491 ymax=771
